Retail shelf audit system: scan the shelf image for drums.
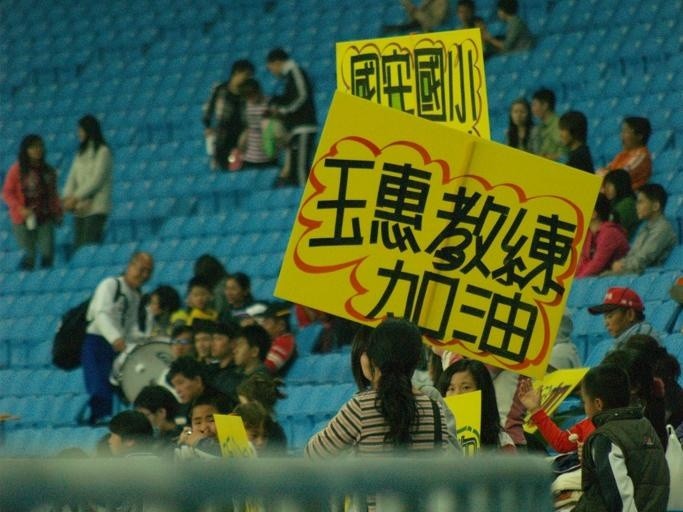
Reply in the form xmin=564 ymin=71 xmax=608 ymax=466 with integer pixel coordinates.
xmin=109 ymin=334 xmax=174 ymax=405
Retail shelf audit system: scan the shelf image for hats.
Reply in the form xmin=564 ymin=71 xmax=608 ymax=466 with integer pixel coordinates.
xmin=255 ymin=302 xmax=292 ymax=321
xmin=586 ymin=286 xmax=645 ymax=315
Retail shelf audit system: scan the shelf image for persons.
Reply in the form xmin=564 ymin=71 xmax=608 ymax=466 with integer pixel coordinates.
xmin=0 ymin=134 xmax=64 ymax=272
xmin=63 ymin=114 xmax=113 ymax=254
xmin=304 ymin=287 xmax=683 ymax=512
xmin=82 ymin=251 xmax=298 ymax=459
xmin=202 ymin=60 xmax=255 ymax=169
xmin=599 ymin=183 xmax=676 ymax=277
xmin=237 ymin=78 xmax=279 ymax=168
xmin=500 ymin=86 xmax=677 ymax=192
xmin=382 ymin=1 xmax=536 ymax=62
xmin=602 ymin=168 xmax=640 ymax=243
xmin=575 ymin=191 xmax=631 ymax=279
xmin=265 ymin=48 xmax=320 ymax=186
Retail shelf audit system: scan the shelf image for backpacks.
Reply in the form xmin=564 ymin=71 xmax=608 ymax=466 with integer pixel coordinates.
xmin=51 ymin=298 xmax=92 ymax=371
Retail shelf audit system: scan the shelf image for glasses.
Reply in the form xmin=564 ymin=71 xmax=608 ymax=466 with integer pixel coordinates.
xmin=168 ymin=337 xmax=195 ymax=346
